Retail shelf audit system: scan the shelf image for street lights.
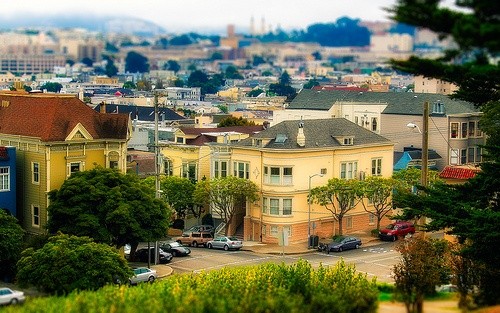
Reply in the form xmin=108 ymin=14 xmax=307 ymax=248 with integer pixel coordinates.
xmin=408 ymin=123 xmax=428 ymax=238
xmin=307 ymin=174 xmax=325 ymax=250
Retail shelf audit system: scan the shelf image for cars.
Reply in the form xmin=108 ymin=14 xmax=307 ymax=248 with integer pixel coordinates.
xmin=158 ymin=241 xmax=192 ymax=257
xmin=0 ymin=287 xmax=25 ymax=307
xmin=327 ymin=235 xmax=362 ymax=252
xmin=206 ymin=235 xmax=244 ymax=251
xmin=124 ymin=243 xmax=132 ymax=261
xmin=135 ymin=247 xmax=174 ymax=264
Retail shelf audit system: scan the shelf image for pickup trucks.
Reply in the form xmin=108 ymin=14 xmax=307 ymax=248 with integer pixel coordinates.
xmin=378 ymin=221 xmax=415 ymax=241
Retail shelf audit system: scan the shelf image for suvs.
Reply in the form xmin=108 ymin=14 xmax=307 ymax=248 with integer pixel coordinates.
xmin=115 ymin=267 xmax=157 ymax=288
xmin=176 ymin=231 xmax=214 ymax=248
xmin=188 ymin=225 xmax=216 ymax=233
